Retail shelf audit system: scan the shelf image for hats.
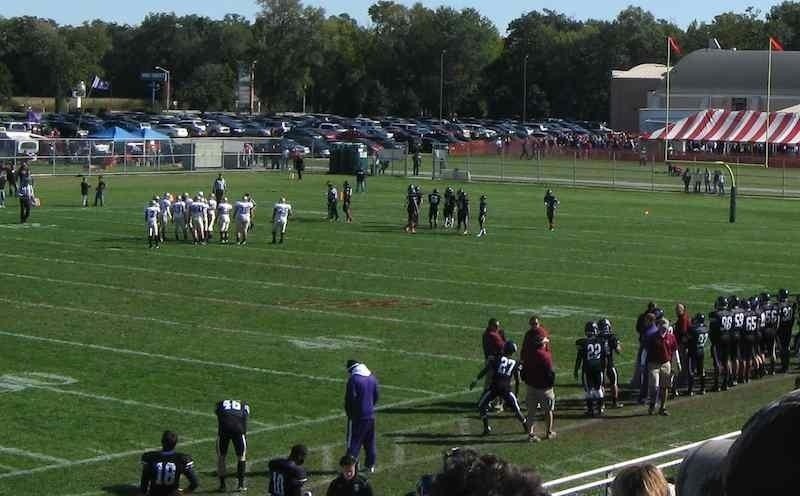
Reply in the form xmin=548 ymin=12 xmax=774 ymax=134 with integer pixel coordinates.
xmin=346 ymin=359 xmax=359 ymax=371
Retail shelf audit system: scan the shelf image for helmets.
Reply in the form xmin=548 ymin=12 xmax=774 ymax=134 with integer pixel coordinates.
xmin=714 ymin=287 xmax=790 ymax=309
xmin=652 ymin=307 xmax=665 ymax=321
xmin=692 ymin=312 xmax=706 ymax=324
xmin=585 ymin=320 xmax=600 ymax=337
xmin=503 ymin=339 xmax=518 ymax=353
xmin=598 ymin=318 xmax=614 ymax=335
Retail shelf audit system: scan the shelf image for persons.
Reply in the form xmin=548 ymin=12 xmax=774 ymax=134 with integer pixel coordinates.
xmin=325 ymin=179 xmax=352 ymax=224
xmin=270 ymin=196 xmax=292 ymax=243
xmin=142 ymin=431 xmax=199 ymax=496
xmin=343 ymin=359 xmax=380 ymax=472
xmin=268 ymin=444 xmax=312 ymax=495
xmin=143 ymin=172 xmax=255 ymax=250
xmin=355 ymin=152 xmax=390 ymax=193
xmin=241 ymin=140 xmax=308 ymax=169
xmin=543 ymin=188 xmax=558 ymax=229
xmin=80 ymin=177 xmax=92 ymax=206
xmin=326 ymin=454 xmax=374 ymax=496
xmin=93 ymin=175 xmax=106 ymax=206
xmin=214 ymin=400 xmax=251 ymax=491
xmin=0 ymin=150 xmax=39 ymax=223
xmin=412 ymin=150 xmax=423 ymax=175
xmin=295 ymin=155 xmax=305 ymax=180
xmin=403 ymin=182 xmax=488 ymax=239
xmin=611 ymin=463 xmax=669 ymax=496
xmin=469 ymin=288 xmax=800 ymax=442
xmin=419 ymin=448 xmax=551 ymax=496
xmin=666 ymin=160 xmax=726 ymax=195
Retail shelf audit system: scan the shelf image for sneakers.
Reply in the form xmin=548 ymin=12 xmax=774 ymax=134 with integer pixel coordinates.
xmin=528 ymin=435 xmax=541 ymax=442
xmin=581 ymin=364 xmax=777 ymax=416
xmin=404 ymin=223 xmax=487 ymax=239
xmin=547 ymin=431 xmax=556 ymax=438
xmin=147 ymin=234 xmax=246 ymax=250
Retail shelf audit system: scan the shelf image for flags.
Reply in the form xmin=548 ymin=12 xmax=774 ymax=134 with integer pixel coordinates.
xmin=92 ymin=78 xmax=109 ymax=90
xmin=670 ymin=36 xmax=680 ymax=52
xmin=771 ymin=38 xmax=780 ymax=51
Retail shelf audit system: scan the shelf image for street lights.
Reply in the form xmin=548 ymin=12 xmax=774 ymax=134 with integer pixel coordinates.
xmin=155 ymin=67 xmax=170 ymax=112
